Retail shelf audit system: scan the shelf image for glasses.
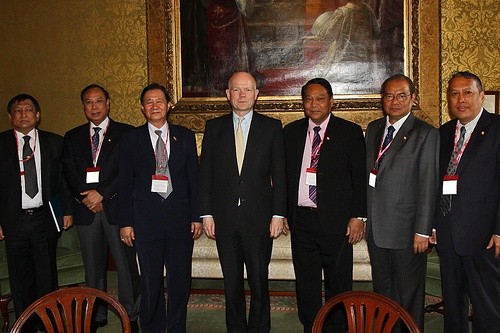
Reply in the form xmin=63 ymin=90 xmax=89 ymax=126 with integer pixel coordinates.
xmin=382 ymin=93 xmax=412 ymax=102
xmin=83 ymin=99 xmax=106 ymax=106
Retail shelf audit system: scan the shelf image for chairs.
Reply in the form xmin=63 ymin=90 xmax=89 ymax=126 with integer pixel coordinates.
xmin=311 ymin=290 xmax=422 ymax=333
xmin=0 ymin=229 xmax=87 ymax=333
xmin=11 ymin=287 xmax=130 ymax=333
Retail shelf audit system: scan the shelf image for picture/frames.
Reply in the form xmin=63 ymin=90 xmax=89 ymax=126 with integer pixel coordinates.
xmin=164 ymin=0 xmax=423 ymax=112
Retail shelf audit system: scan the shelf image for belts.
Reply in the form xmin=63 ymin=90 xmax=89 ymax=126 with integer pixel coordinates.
xmin=22 ymin=205 xmax=45 ymax=216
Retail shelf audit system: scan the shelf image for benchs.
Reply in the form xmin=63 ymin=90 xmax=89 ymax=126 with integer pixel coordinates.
xmin=134 ymin=225 xmax=375 ymax=281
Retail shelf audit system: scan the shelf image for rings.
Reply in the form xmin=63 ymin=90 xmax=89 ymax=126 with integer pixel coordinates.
xmin=121 ymin=237 xmax=124 ymax=241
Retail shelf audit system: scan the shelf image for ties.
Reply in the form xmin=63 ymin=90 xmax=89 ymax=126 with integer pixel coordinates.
xmin=378 ymin=126 xmax=396 ymax=172
xmin=235 ymin=117 xmax=245 ymax=176
xmin=154 ymin=129 xmax=173 ymax=200
xmin=90 ymin=127 xmax=102 ymax=162
xmin=439 ymin=126 xmax=466 ymax=217
xmin=22 ymin=136 xmax=38 ymax=198
xmin=308 ymin=126 xmax=321 ymax=206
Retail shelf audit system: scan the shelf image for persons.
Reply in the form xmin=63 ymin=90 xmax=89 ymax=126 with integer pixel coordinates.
xmin=365 ymin=73 xmax=461 ymax=333
xmin=118 ymin=83 xmax=202 ymax=333
xmin=283 ymin=78 xmax=365 ymax=333
xmin=0 ymin=94 xmax=74 ymax=333
xmin=62 ymin=85 xmax=141 ymax=333
xmin=428 ymin=70 xmax=500 ymax=333
xmin=198 ymin=71 xmax=285 ymax=333
